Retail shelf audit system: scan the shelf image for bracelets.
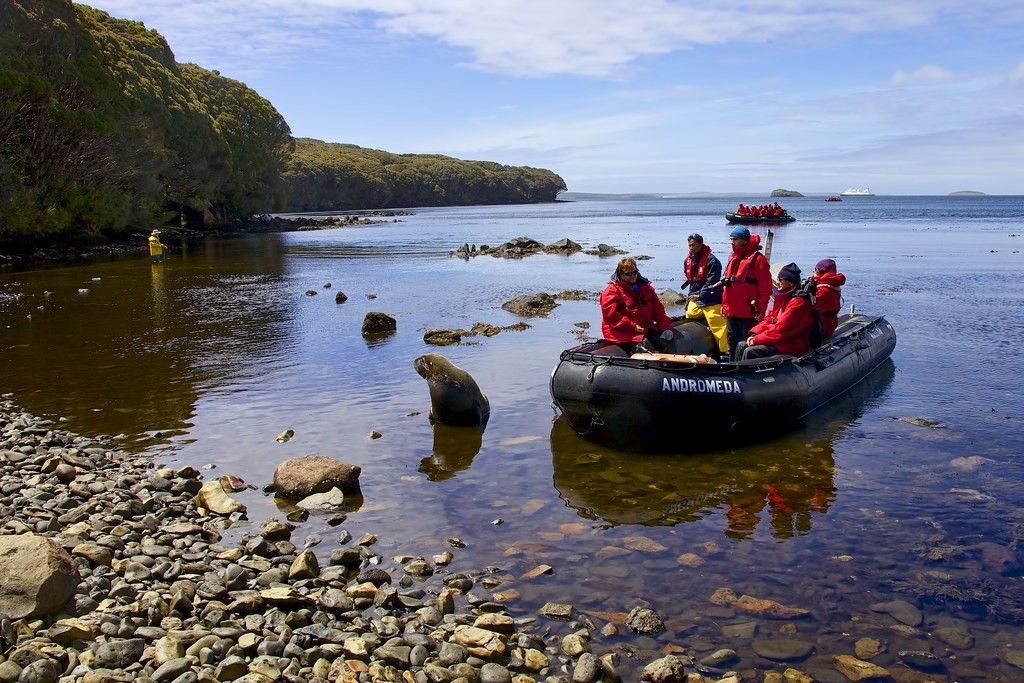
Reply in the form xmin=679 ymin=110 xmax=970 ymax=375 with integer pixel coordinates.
xmin=697 ymin=294 xmax=699 ymax=301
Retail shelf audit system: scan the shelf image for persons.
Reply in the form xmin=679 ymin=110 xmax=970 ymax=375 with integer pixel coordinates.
xmin=735 ymin=262 xmax=813 ymax=363
xmin=684 ymin=233 xmax=731 ymax=363
xmin=807 ymin=259 xmax=846 ymax=341
xmin=737 ymin=202 xmax=784 ymax=217
xmin=724 ymin=454 xmax=834 ymax=545
xmin=723 ymin=227 xmax=773 ymax=363
xmin=600 ymin=258 xmax=677 ymax=355
xmin=149 ymin=229 xmax=167 ymax=264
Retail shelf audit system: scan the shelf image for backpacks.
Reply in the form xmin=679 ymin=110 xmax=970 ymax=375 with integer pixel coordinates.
xmin=781 ymin=296 xmax=823 ymax=349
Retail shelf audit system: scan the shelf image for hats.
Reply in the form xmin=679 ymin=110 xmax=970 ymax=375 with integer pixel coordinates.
xmin=778 ymin=262 xmax=801 ymax=285
xmin=730 ymin=226 xmax=750 ymax=241
xmin=151 ymin=229 xmax=162 ymax=236
xmin=816 ymin=259 xmax=835 ymax=274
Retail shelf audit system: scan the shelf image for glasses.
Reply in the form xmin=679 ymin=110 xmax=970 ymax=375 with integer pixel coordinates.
xmin=688 ymin=234 xmax=702 ymax=240
xmin=779 ymin=277 xmax=783 ymax=282
xmin=815 ymin=267 xmax=816 ymax=271
xmin=621 ymin=269 xmax=639 ymax=276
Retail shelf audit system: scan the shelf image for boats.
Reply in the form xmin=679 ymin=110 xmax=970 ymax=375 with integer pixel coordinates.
xmin=549 ymin=303 xmax=897 ymax=440
xmin=840 ymin=188 xmax=875 ymax=196
xmin=825 ymin=198 xmax=843 ymax=201
xmin=725 ymin=212 xmax=796 ymax=223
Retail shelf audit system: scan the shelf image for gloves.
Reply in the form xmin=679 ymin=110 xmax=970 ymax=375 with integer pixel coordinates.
xmin=662 ymin=330 xmax=673 ymax=341
